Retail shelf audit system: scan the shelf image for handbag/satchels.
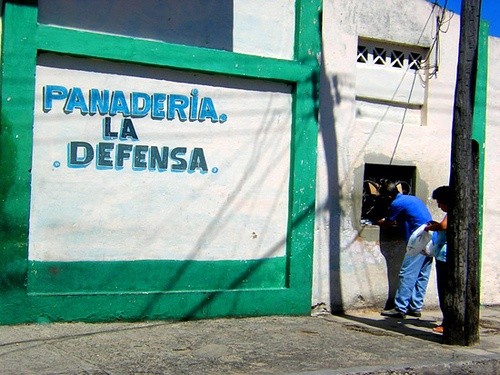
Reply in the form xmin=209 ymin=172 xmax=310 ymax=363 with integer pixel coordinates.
xmin=407 ymin=223 xmax=432 ymax=256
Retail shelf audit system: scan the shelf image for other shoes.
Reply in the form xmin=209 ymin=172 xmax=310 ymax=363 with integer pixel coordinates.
xmin=433 ymin=326 xmax=443 ymax=332
xmin=405 ymin=307 xmax=421 ymax=317
xmin=381 ymin=308 xmax=406 ymax=318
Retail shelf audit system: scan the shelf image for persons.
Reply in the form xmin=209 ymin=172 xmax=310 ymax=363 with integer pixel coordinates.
xmin=373 ymin=181 xmax=434 ymax=319
xmin=423 ymin=185 xmax=450 ymax=334
